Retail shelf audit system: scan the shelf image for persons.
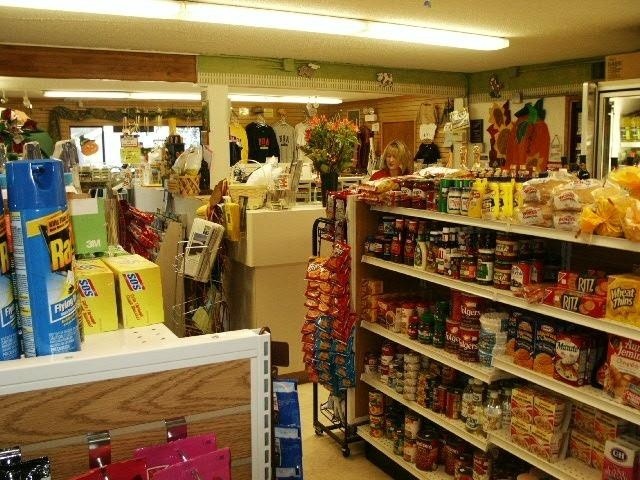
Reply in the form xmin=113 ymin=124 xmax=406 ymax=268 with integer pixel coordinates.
xmin=369 ymin=138 xmax=414 ymax=181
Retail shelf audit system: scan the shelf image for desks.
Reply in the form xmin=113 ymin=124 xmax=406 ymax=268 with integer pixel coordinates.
xmin=131 ymin=182 xmax=168 ymax=214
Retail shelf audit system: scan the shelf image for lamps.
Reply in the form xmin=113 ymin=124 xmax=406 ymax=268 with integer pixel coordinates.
xmin=121 ymin=106 xmax=163 ymax=133
xmin=227 ymin=93 xmax=343 ymax=106
xmin=0 ymin=90 xmax=33 ymax=109
xmin=41 ymin=89 xmax=205 ymax=101
xmin=0 ymin=0 xmax=510 ymax=52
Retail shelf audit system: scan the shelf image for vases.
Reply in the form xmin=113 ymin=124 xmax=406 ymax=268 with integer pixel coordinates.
xmin=320 ymin=171 xmax=339 ymax=207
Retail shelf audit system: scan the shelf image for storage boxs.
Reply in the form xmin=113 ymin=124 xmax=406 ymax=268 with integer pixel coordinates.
xmin=73 ymin=258 xmax=119 ymax=336
xmin=68 ymin=197 xmax=109 ymax=255
xmin=100 ymin=254 xmax=165 ymax=330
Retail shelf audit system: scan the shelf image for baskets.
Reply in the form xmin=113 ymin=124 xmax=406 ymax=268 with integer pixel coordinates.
xmin=164 ymin=172 xmax=201 ymax=197
xmin=228 ymin=159 xmax=267 ymax=210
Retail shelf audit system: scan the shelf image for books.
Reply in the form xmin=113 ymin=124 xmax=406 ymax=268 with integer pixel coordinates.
xmin=180 ymin=217 xmax=225 ymax=283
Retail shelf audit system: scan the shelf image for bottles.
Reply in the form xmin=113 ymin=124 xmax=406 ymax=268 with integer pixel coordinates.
xmin=626 ymin=157 xmax=634 ymax=166
xmin=460 ymin=186 xmax=470 ymax=216
xmin=460 ymin=377 xmax=512 ymax=439
xmin=446 ymin=187 xmax=461 ymax=215
xmin=611 ymin=157 xmax=618 ymax=171
xmin=560 ymin=157 xmax=567 ymax=169
xmin=473 ymin=164 xmax=539 ymax=182
xmin=577 ymin=154 xmax=590 ymax=179
xmin=413 ymin=226 xmax=474 ymax=273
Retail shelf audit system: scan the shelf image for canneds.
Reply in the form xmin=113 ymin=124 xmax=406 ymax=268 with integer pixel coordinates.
xmin=364 ymin=340 xmax=464 ymax=420
xmin=475 ymin=231 xmax=545 ymax=291
xmin=368 ymin=388 xmax=499 ymax=480
xmin=400 ymin=180 xmax=434 ymax=209
xmin=445 ymin=291 xmax=485 ymax=363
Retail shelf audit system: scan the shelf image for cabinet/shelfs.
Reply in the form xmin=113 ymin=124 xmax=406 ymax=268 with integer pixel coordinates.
xmin=345 ymin=192 xmax=640 ymax=480
xmin=78 ymin=164 xmax=121 ymax=199
xmin=231 ymin=162 xmax=322 ymax=203
xmin=168 ymin=240 xmax=234 ymax=339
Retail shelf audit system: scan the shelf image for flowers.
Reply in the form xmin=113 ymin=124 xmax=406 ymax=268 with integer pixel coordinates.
xmin=296 ymin=108 xmax=363 ymax=179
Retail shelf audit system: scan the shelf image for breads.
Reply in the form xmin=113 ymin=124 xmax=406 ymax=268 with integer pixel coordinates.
xmin=521 ymin=167 xmax=640 ymax=241
xmin=505 ymin=338 xmax=554 ymax=376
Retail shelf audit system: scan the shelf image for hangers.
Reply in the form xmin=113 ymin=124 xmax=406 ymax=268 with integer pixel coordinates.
xmin=230 ymin=110 xmax=313 ymax=126
